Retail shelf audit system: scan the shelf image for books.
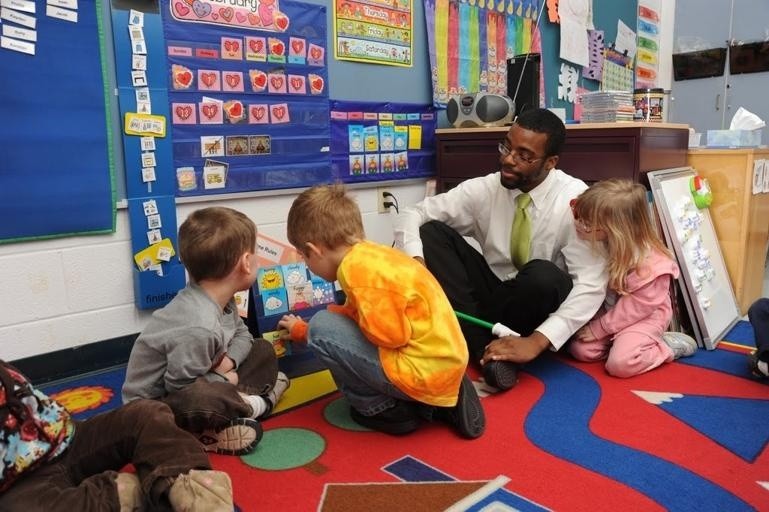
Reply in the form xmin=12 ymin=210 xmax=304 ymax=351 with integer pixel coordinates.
xmin=581 ymin=91 xmax=635 ymax=124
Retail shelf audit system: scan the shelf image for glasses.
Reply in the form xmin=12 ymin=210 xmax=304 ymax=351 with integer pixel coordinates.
xmin=574 ymin=222 xmax=600 ymax=233
xmin=497 ymin=142 xmax=543 ymax=166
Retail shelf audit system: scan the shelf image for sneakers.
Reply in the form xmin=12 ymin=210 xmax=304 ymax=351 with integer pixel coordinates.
xmin=349 ymin=401 xmax=420 ymax=437
xmin=485 ymin=360 xmax=516 ymax=391
xmin=265 ymin=371 xmax=289 ymax=412
xmin=449 ymin=373 xmax=485 ymax=438
xmin=169 ymin=470 xmax=234 ymax=511
xmin=662 ymin=332 xmax=698 ymax=359
xmin=116 ymin=472 xmax=142 ymax=512
xmin=196 ymin=418 xmax=263 ymax=455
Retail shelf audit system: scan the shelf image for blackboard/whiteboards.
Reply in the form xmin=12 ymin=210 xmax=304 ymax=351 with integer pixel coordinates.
xmin=647 ymin=166 xmax=742 ymax=351
xmin=101 ymin=0 xmax=639 ymax=211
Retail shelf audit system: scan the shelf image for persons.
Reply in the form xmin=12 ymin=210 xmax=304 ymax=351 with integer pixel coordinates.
xmin=748 ymin=297 xmax=769 ymax=380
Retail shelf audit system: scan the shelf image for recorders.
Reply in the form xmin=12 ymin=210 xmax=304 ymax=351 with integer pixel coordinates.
xmin=446 ymin=91 xmax=518 ymax=128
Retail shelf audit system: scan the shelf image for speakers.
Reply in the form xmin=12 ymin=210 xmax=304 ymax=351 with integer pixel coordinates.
xmin=508 ymin=53 xmax=542 ymax=113
